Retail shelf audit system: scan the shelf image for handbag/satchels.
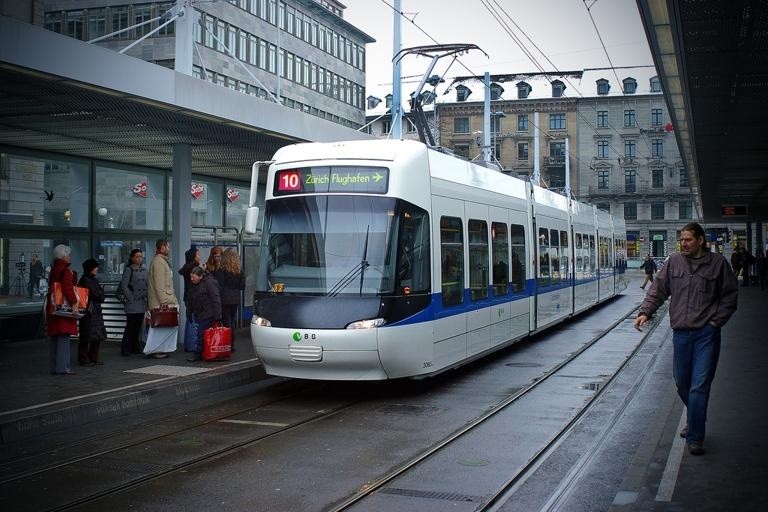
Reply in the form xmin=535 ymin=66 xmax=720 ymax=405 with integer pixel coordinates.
xmin=202 ymin=321 xmax=232 ymax=361
xmin=151 ymin=305 xmax=179 ymax=327
xmin=185 ymin=313 xmax=199 ymax=352
xmin=50 ymin=281 xmax=90 ymax=318
xmin=39 ymin=278 xmax=48 ymax=294
xmin=115 ymin=282 xmax=133 ymax=305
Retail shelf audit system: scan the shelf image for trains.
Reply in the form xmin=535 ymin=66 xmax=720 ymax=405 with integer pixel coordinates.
xmin=244 ymin=134 xmax=629 ymax=387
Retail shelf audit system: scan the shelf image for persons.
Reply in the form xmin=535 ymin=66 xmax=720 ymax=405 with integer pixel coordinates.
xmin=730 ymin=245 xmax=768 ymax=294
xmin=25 ymin=254 xmax=44 ymax=299
xmin=632 ymin=220 xmax=738 ymax=455
xmin=42 ymin=237 xmax=245 ymax=374
xmin=614 ymin=254 xmax=628 ymax=289
xmin=639 ymin=252 xmax=657 ymax=289
xmin=442 ymin=264 xmax=463 ymax=304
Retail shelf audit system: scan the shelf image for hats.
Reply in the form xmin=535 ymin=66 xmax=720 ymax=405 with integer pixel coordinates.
xmin=82 ymin=258 xmax=99 ymax=274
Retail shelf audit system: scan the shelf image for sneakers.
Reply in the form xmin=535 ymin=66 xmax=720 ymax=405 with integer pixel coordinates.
xmin=121 ymin=350 xmax=142 ymax=357
xmin=186 ymin=355 xmax=201 ymax=361
xmin=66 ymin=367 xmax=77 ymax=374
xmin=80 ymin=362 xmax=104 ymax=366
xmin=152 ymin=353 xmax=167 ymax=358
xmin=688 ymin=443 xmax=704 ymax=455
xmin=680 ymin=425 xmax=689 ymax=437
